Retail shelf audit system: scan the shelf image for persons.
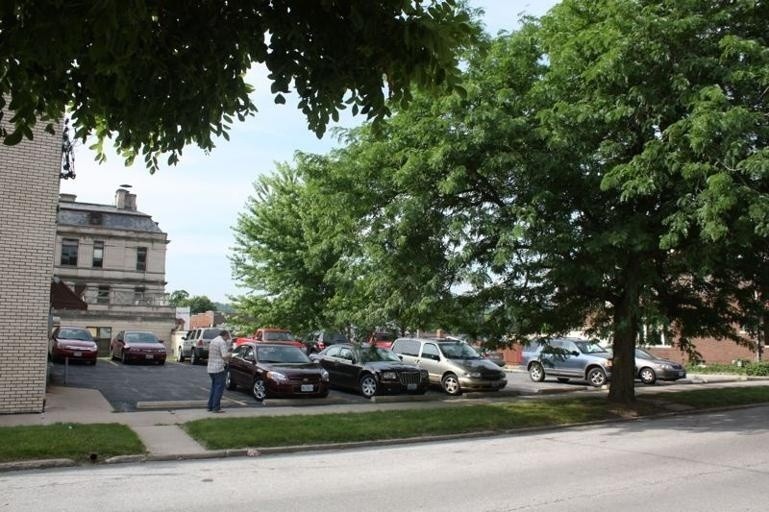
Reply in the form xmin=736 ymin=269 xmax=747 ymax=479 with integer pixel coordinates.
xmin=206 ymin=329 xmax=233 ymax=413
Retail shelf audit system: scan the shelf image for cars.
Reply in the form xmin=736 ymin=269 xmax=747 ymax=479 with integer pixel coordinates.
xmin=110 ymin=329 xmax=166 ymax=367
xmin=602 ymin=345 xmax=686 ymax=384
xmin=48 ymin=325 xmax=98 ymax=366
xmin=225 ymin=326 xmax=507 ymax=401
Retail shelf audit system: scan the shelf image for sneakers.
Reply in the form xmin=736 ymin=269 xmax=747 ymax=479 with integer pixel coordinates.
xmin=208 ymin=408 xmax=225 ymax=413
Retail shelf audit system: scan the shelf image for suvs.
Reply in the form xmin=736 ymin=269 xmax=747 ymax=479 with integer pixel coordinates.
xmin=519 ymin=335 xmax=613 ymax=387
xmin=176 ymin=326 xmax=233 ymax=367
